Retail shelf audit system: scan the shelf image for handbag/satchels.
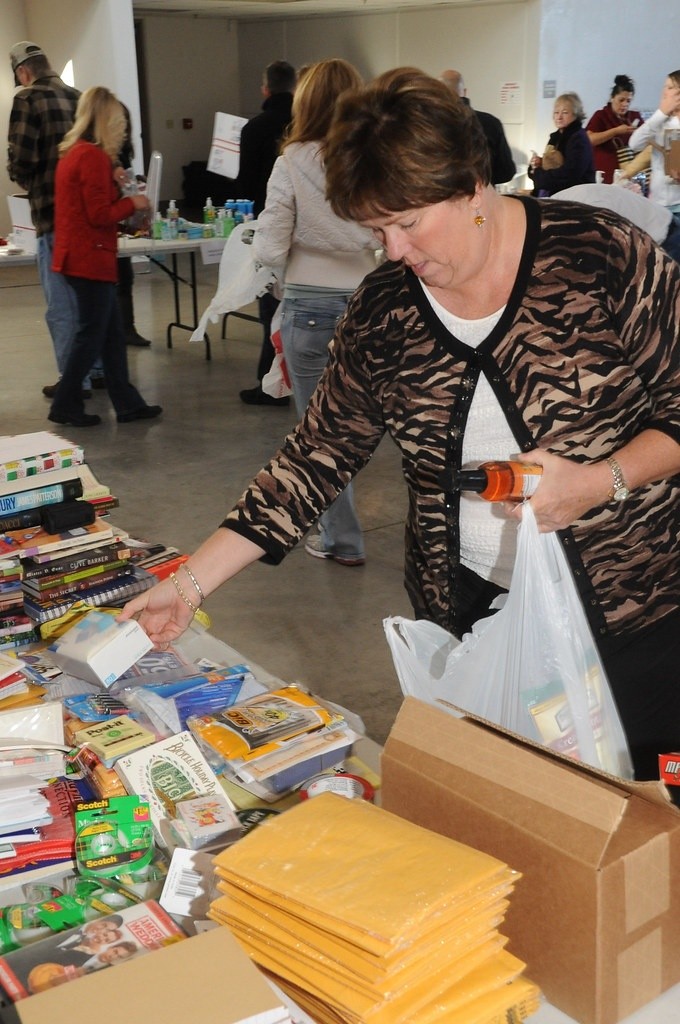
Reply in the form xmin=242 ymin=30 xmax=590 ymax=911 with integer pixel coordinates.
xmin=384 ymin=499 xmax=638 ymax=781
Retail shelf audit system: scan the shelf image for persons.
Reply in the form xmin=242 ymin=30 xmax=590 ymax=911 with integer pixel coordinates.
xmin=628 ymin=70 xmax=680 ymax=217
xmin=251 ymin=59 xmax=386 ymax=564
xmin=586 ymin=75 xmax=645 ymax=184
xmin=114 ymin=102 xmax=150 ymax=344
xmin=119 ymin=68 xmax=680 ymax=805
xmin=437 ymin=70 xmax=516 ymax=189
xmin=76 ymin=930 xmax=122 ymax=956
xmin=61 ymin=942 xmax=139 ymax=976
xmin=6 ymin=42 xmax=105 ymax=397
xmin=51 ymin=914 xmax=123 ymax=950
xmin=519 ymin=93 xmax=595 ymax=200
xmin=549 ymin=183 xmax=680 ymax=271
xmin=47 ymin=87 xmax=164 ymax=429
xmin=236 ymin=62 xmax=298 ymax=407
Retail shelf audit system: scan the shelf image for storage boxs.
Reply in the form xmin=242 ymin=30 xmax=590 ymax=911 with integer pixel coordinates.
xmin=46 ymin=607 xmax=155 ymax=689
xmin=494 ymin=173 xmax=527 ymax=196
xmin=113 ymin=729 xmax=246 ymax=859
xmin=647 ymin=128 xmax=680 ymax=179
xmin=7 ymin=192 xmax=37 ymax=252
xmin=376 ymin=694 xmax=680 ymax=1024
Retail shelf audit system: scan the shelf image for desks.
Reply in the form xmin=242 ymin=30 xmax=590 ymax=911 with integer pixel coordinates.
xmin=170 ymin=619 xmax=384 ymax=809
xmin=0 ymin=237 xmax=263 ymax=362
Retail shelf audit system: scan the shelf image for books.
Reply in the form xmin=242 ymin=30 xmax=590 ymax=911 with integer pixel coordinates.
xmin=0 ymin=429 xmax=538 ymax=1024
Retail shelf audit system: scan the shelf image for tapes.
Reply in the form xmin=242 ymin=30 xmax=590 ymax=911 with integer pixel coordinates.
xmin=300 ymin=774 xmax=375 ymax=804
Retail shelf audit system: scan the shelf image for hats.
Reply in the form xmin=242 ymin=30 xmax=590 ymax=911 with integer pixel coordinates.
xmin=8 ymin=40 xmax=46 ymax=87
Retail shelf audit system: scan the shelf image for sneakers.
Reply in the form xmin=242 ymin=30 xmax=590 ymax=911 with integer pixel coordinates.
xmin=305 ymin=522 xmax=367 ymax=567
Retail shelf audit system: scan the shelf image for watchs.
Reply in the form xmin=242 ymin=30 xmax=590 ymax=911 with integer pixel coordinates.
xmin=603 ymin=458 xmax=630 ymax=504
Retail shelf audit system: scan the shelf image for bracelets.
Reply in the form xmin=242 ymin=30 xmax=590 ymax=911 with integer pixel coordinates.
xmin=179 ymin=562 xmax=204 ymax=603
xmin=170 ymin=572 xmax=198 ymax=613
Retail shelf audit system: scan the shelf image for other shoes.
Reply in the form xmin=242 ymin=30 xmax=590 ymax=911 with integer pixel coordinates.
xmin=238 ymin=385 xmax=292 ymax=405
xmin=90 ymin=378 xmax=108 ymax=390
xmin=42 ymin=382 xmax=94 ymax=399
xmin=117 ymin=402 xmax=162 ymax=422
xmin=126 ymin=333 xmax=152 ymax=347
xmin=47 ymin=408 xmax=102 ymax=427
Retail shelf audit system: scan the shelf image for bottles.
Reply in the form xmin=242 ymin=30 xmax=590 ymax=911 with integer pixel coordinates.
xmin=153 ymin=212 xmax=162 ymax=237
xmin=441 ymin=461 xmax=542 ymax=501
xmin=224 ymin=199 xmax=254 ymax=215
xmin=162 ymin=219 xmax=177 ymax=238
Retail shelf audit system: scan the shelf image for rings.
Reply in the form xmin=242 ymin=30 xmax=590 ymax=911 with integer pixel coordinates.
xmin=119 ymin=175 xmax=124 ymax=179
